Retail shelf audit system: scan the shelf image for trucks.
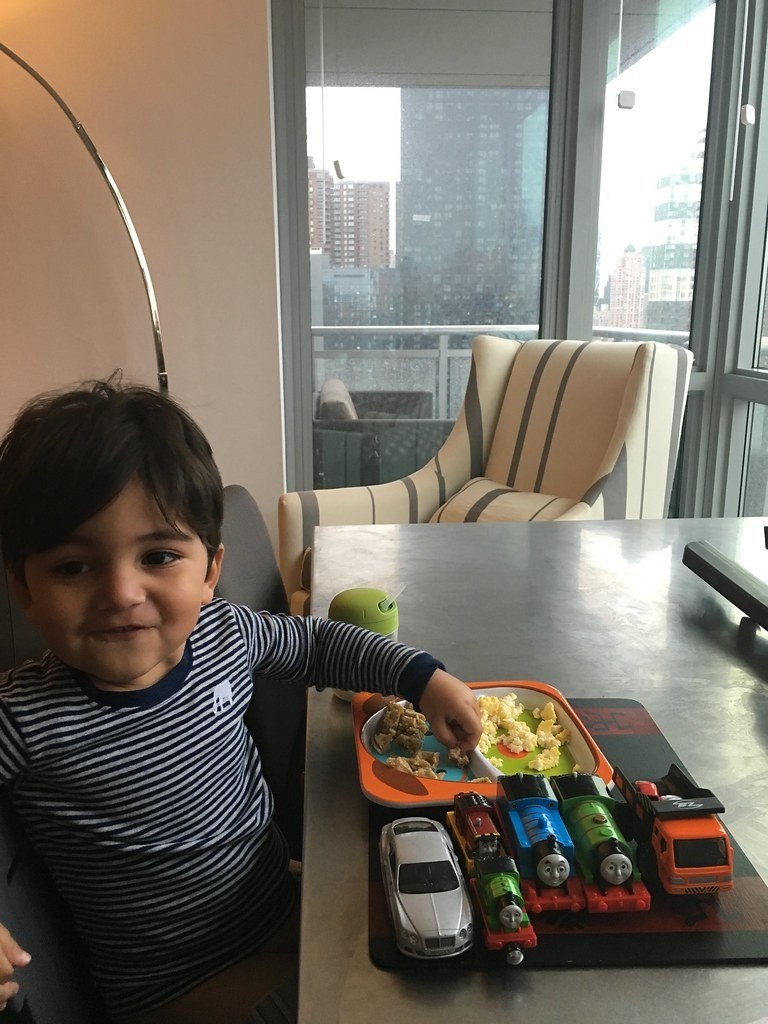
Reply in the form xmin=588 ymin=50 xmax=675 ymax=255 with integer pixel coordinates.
xmin=606 ymin=761 xmax=735 ymax=898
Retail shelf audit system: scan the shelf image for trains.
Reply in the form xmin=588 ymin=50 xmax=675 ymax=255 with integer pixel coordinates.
xmin=494 ymin=770 xmax=587 ymax=927
xmin=446 ymin=789 xmax=540 ymax=970
xmin=550 ymin=767 xmax=652 ymax=918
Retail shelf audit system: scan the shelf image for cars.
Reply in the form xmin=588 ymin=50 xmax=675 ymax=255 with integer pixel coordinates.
xmin=378 ymin=815 xmax=475 ymax=963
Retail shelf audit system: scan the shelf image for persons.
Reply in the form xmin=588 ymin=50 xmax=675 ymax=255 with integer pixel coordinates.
xmin=0 ymin=367 xmax=480 ymax=1024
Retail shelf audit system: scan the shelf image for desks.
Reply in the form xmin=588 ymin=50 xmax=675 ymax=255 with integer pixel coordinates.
xmin=299 ymin=516 xmax=768 ymax=1024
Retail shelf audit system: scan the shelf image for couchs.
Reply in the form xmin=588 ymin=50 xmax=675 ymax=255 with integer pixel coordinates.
xmin=280 ymin=333 xmax=694 ymax=631
xmin=314 ymin=378 xmax=456 ymax=492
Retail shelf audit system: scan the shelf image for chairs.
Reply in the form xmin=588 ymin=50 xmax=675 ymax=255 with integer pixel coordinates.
xmin=0 ymin=484 xmax=290 ymax=1024
